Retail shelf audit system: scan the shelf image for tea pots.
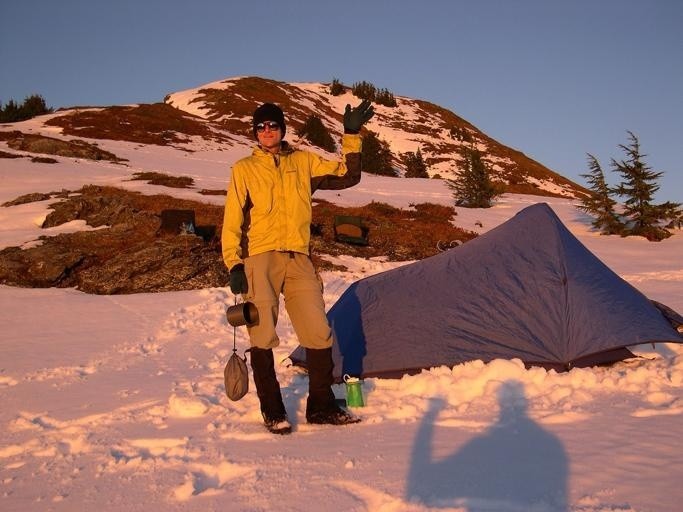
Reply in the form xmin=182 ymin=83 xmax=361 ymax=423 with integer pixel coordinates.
xmin=343 ymin=374 xmax=365 ymax=408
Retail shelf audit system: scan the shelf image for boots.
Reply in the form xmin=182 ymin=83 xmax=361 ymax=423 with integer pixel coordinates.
xmin=249 ymin=347 xmax=292 ymax=433
xmin=306 ymin=348 xmax=361 ymax=425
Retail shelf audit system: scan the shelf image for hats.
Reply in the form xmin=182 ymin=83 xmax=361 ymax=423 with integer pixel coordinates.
xmin=253 ymin=103 xmax=285 ymax=130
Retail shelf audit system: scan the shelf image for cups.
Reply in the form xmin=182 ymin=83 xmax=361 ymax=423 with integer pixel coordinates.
xmin=226 ymin=301 xmax=259 ymax=327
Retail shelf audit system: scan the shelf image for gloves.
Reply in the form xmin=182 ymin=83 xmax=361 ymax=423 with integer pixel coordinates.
xmin=229 ymin=264 xmax=248 ymax=294
xmin=343 ymin=99 xmax=374 ymax=135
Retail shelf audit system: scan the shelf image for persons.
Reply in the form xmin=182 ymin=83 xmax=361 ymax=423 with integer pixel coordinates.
xmin=220 ymin=98 xmax=375 ymax=437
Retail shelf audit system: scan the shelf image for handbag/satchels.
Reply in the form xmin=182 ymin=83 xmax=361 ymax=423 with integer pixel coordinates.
xmin=225 ymin=353 xmax=249 ymax=401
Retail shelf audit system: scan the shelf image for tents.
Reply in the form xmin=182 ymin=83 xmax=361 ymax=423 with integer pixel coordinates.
xmin=284 ymin=200 xmax=683 ymax=383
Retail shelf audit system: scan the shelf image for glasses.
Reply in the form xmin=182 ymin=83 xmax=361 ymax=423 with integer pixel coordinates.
xmin=256 ymin=121 xmax=279 ymax=129
